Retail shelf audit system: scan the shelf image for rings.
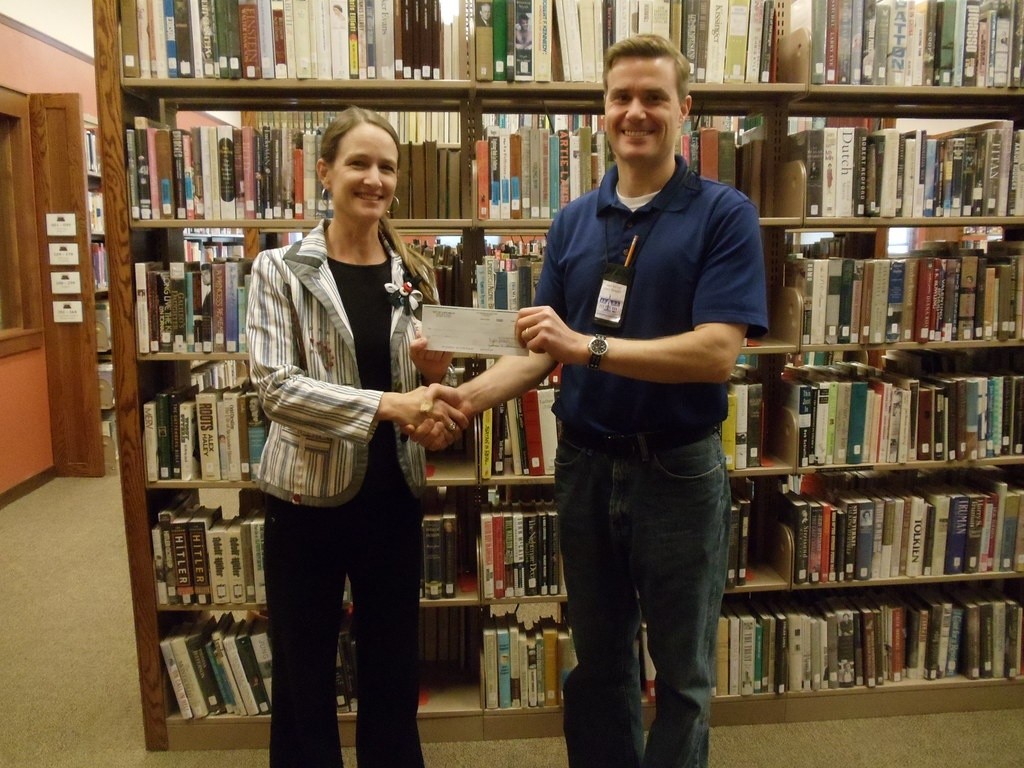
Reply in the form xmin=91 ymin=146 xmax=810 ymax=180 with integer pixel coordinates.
xmin=448 ymin=422 xmax=458 ymax=432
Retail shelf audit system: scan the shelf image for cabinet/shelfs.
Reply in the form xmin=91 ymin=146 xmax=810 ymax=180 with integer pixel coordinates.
xmin=29 ymin=92 xmax=261 ymax=478
xmin=91 ymin=2 xmax=1024 ymax=754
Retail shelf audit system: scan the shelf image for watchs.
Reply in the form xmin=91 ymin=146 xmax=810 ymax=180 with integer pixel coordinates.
xmin=585 ymin=333 xmax=608 ymax=370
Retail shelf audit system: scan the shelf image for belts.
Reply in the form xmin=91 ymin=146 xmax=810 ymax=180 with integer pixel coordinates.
xmin=561 ymin=418 xmax=720 ymax=458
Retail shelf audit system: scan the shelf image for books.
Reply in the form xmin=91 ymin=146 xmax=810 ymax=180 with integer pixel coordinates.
xmin=121 ymin=1 xmax=461 ymax=79
xmin=764 ymin=462 xmax=1024 ymax=584
xmin=481 ymin=231 xmax=1024 ymax=344
xmin=82 ymin=125 xmax=122 ymax=479
xmin=474 ymin=99 xmax=1023 ymax=218
xmin=769 ymin=347 xmax=1024 ymax=465
xmin=477 ymin=1 xmax=777 ymax=83
xmin=713 ymin=580 xmax=1024 ymax=699
xmin=155 ymin=610 xmax=367 ymax=719
xmin=477 ymin=484 xmax=781 ymax=599
xmin=416 ymin=606 xmax=660 ymax=709
xmin=789 ymin=0 xmax=1022 ymax=89
xmin=477 ymin=349 xmax=769 ymax=479
xmin=134 ymin=227 xmax=485 ymax=358
xmin=151 ymin=488 xmax=476 ymax=607
xmin=125 ymin=106 xmax=464 ymax=221
xmin=139 ymin=358 xmax=472 ymax=484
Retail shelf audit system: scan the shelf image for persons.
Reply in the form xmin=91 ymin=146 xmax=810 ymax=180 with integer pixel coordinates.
xmin=245 ymin=105 xmax=476 ymax=768
xmin=403 ymin=32 xmax=769 ymax=768
